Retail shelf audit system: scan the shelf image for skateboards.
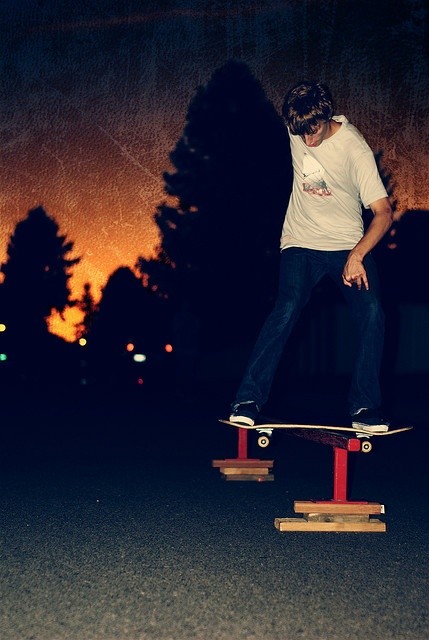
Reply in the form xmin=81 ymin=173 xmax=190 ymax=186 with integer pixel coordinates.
xmin=218 ymin=419 xmax=414 ymax=453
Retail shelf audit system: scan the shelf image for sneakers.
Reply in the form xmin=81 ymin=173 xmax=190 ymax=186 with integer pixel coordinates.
xmin=352 ymin=408 xmax=389 ymax=432
xmin=229 ymin=403 xmax=258 ymax=426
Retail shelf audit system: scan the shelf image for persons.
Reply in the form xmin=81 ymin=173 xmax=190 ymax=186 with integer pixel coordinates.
xmin=229 ymin=78 xmax=394 ymax=433
